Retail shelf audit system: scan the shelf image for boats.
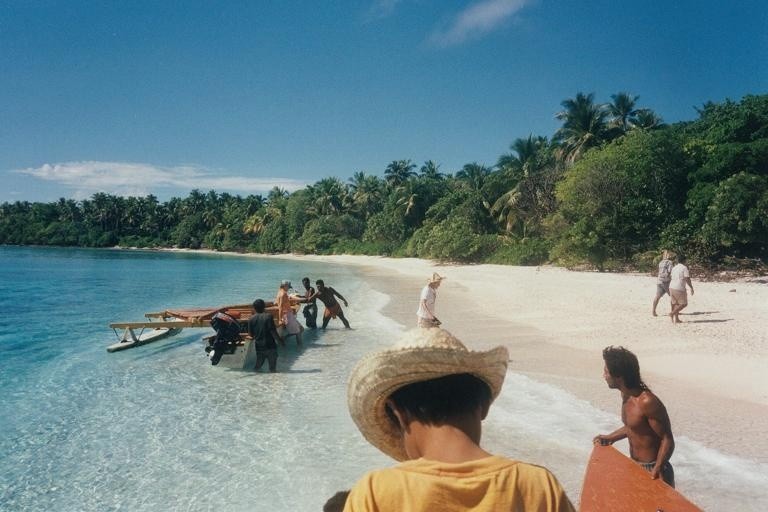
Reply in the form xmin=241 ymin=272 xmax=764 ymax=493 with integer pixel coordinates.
xmin=165 ymin=291 xmax=301 ymax=328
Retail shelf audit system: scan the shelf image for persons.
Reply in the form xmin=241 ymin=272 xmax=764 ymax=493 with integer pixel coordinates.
xmin=669 ymin=254 xmax=694 ymax=324
xmin=286 ymin=282 xmax=297 ymax=295
xmin=594 ymin=345 xmax=675 ymax=490
xmin=248 ymin=299 xmax=284 ymax=373
xmin=296 ymin=277 xmax=318 ymax=329
xmin=652 ymin=250 xmax=674 ymax=317
xmin=343 ymin=325 xmax=576 ymax=512
xmin=277 ymin=280 xmax=304 ymax=345
xmin=307 ymin=280 xmax=350 ymax=329
xmin=417 ymin=273 xmax=445 ymax=329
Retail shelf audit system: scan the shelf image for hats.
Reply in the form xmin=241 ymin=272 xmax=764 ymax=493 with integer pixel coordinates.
xmin=282 ymin=280 xmax=293 ymax=288
xmin=664 ymin=249 xmax=676 ymax=259
xmin=347 ymin=327 xmax=510 ymax=462
xmin=429 ymin=272 xmax=445 ymax=284
